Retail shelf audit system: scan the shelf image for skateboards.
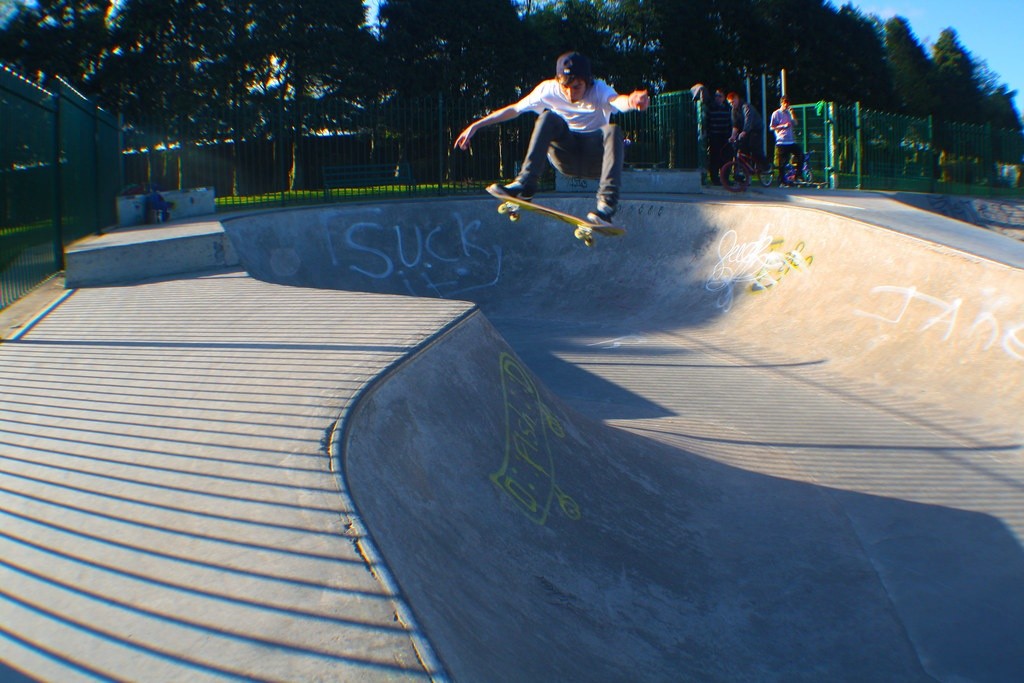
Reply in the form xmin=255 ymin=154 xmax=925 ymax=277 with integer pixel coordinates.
xmin=484 ymin=185 xmax=629 ymax=248
xmin=792 ymin=181 xmax=830 ymax=190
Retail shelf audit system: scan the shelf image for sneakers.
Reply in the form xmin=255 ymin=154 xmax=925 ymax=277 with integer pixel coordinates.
xmin=587 ymin=200 xmax=614 ymax=225
xmin=492 ymin=177 xmax=534 ymax=201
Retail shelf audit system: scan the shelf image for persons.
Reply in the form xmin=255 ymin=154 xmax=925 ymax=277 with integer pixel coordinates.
xmin=624 ymin=130 xmax=641 ymax=144
xmin=707 ymin=89 xmax=733 ymax=186
xmin=453 ymin=52 xmax=648 ymax=226
xmin=770 ymin=95 xmax=804 ymax=187
xmin=145 ymin=184 xmax=167 ymax=224
xmin=726 ymin=92 xmax=764 ymax=182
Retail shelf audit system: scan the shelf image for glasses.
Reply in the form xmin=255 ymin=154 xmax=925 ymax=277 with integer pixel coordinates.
xmin=715 ymin=94 xmax=724 ymax=98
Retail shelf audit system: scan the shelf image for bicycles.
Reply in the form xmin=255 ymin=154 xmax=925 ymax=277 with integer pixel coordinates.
xmin=719 ymin=134 xmax=775 ymax=193
xmin=782 ymin=149 xmax=816 ymax=185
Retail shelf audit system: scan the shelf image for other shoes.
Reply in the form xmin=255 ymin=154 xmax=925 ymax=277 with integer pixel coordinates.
xmin=712 ymin=178 xmax=722 ymax=185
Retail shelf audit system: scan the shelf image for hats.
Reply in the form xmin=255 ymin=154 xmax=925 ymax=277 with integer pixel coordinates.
xmin=556 ymin=52 xmax=590 ymax=75
